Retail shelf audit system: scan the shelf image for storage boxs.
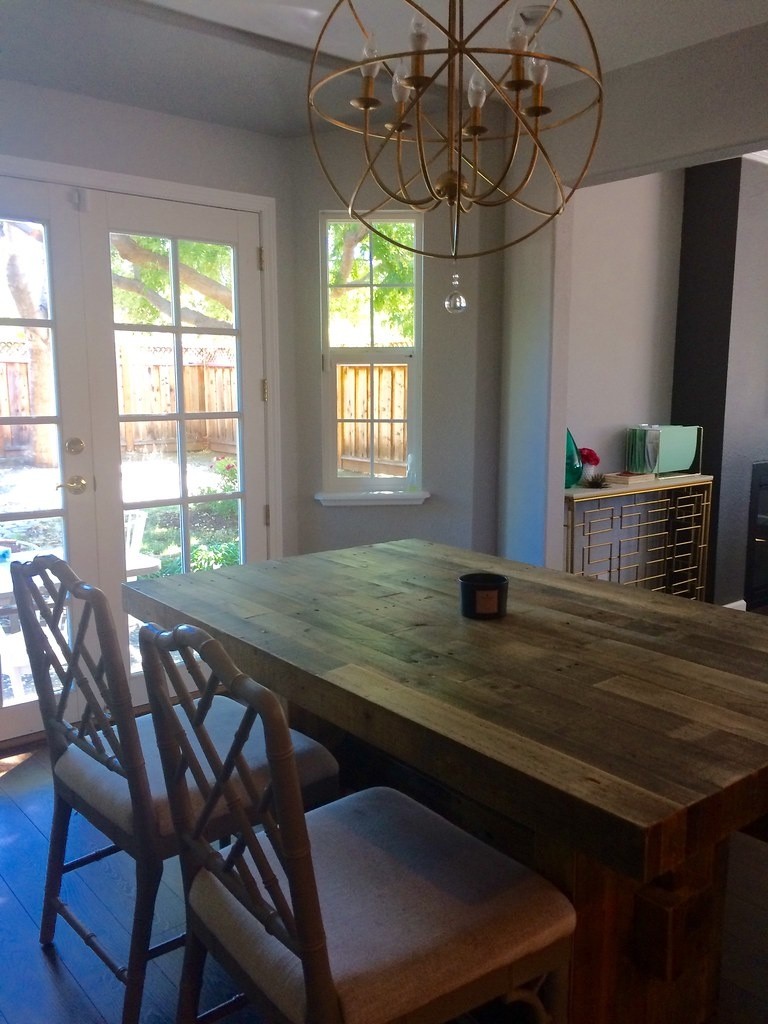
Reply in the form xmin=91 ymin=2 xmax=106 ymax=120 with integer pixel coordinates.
xmin=603 ymin=471 xmax=656 ymax=483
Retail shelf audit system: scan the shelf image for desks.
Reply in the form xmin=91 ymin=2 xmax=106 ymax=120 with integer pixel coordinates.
xmin=122 ymin=539 xmax=768 ymax=1024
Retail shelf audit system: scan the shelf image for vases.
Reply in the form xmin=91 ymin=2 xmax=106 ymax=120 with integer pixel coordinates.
xmin=575 ymin=462 xmax=595 ymax=483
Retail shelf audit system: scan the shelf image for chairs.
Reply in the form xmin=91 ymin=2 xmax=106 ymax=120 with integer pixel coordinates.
xmin=138 ymin=619 xmax=588 ymax=1024
xmin=10 ymin=554 xmax=336 ymax=1024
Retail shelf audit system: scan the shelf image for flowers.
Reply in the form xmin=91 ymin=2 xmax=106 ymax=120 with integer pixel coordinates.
xmin=579 ymin=448 xmax=600 ymax=466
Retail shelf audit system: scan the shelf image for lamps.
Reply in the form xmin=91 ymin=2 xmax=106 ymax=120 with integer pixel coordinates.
xmin=307 ymin=0 xmax=603 ymax=312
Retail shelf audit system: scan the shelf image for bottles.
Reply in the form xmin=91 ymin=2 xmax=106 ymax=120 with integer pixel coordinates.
xmin=404 ymin=453 xmax=420 ymax=493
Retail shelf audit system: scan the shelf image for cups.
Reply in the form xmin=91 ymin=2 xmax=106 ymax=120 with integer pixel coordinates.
xmin=458 ymin=573 xmax=509 ymax=619
